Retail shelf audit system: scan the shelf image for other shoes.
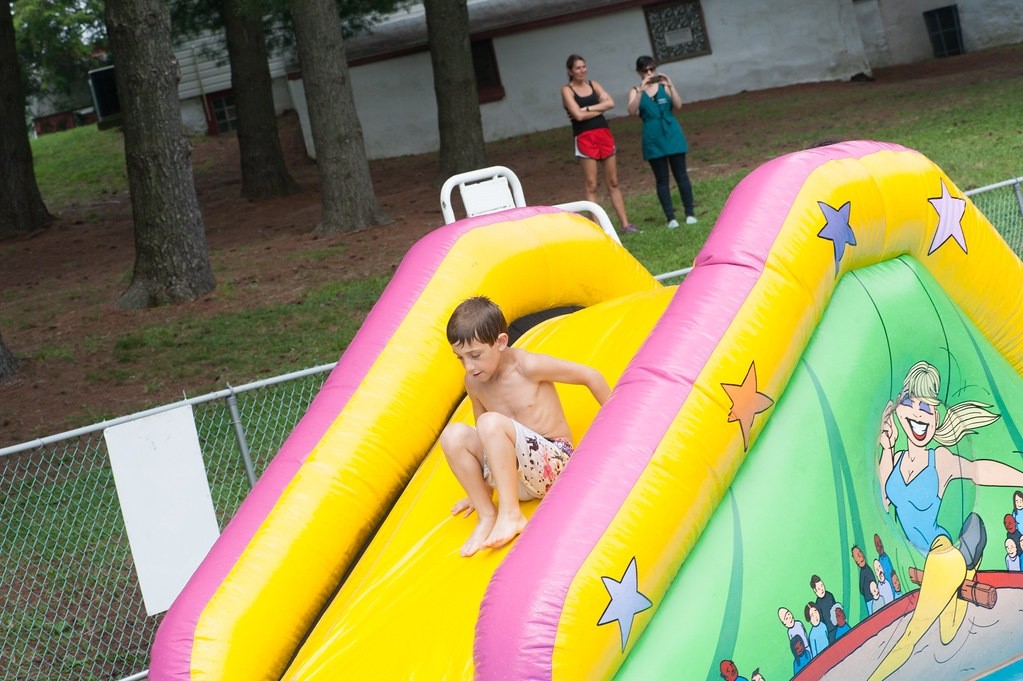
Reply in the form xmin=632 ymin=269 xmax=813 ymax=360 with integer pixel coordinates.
xmin=669 ymin=220 xmax=679 ymax=228
xmin=619 ymin=224 xmax=640 ymax=233
xmin=686 ymin=216 xmax=697 ymax=225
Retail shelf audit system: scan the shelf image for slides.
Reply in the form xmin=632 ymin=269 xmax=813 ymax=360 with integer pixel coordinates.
xmin=150 ymin=141 xmax=1022 ymax=681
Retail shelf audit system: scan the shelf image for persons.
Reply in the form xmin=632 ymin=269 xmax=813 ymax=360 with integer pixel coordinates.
xmin=562 ymin=54 xmax=640 ymax=233
xmin=440 ymin=295 xmax=611 ymax=557
xmin=627 ymin=56 xmax=697 ymax=228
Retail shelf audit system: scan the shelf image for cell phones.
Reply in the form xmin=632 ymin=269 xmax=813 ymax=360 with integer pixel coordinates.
xmin=646 ymin=75 xmax=662 ymax=84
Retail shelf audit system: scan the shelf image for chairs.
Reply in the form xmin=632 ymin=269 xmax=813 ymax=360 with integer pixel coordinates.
xmin=439 ymin=165 xmax=624 ymax=247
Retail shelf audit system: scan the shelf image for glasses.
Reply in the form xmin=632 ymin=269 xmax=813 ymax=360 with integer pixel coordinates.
xmin=641 ymin=64 xmax=656 ymax=73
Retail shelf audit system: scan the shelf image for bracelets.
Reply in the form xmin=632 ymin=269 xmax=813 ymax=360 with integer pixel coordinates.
xmin=586 ymin=105 xmax=589 ymax=111
